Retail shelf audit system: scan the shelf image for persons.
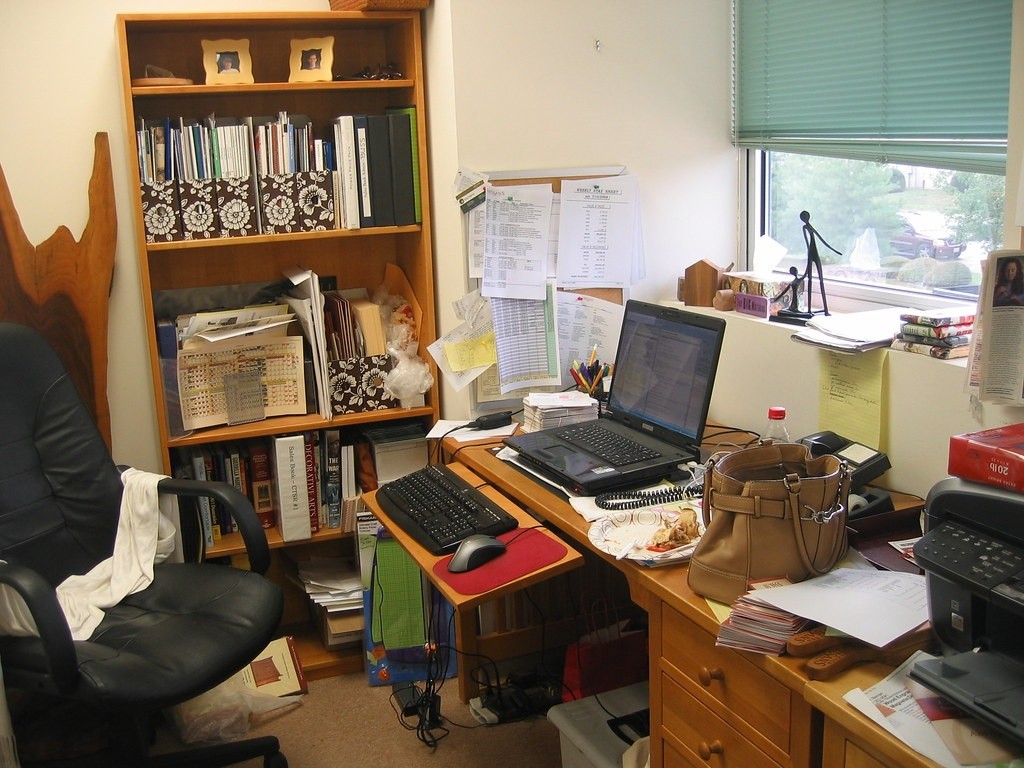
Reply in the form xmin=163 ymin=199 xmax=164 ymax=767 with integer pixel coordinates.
xmin=306 ymin=53 xmax=318 ymax=69
xmin=993 ymin=259 xmax=1024 ymax=306
xmin=219 ymin=58 xmax=238 ymax=74
xmin=773 ymin=211 xmax=843 ymax=319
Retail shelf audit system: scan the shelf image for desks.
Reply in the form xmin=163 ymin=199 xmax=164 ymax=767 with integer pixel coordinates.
xmin=362 ymin=420 xmax=1024 ymax=768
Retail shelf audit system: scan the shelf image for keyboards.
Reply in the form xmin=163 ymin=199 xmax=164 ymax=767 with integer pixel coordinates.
xmin=375 ymin=463 xmax=518 ymax=556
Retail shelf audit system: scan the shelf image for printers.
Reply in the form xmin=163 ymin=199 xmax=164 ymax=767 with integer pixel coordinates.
xmin=906 ymin=476 xmax=1024 ymax=747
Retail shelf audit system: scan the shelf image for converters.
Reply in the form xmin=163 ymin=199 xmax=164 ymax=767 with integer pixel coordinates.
xmin=391 ymin=681 xmax=424 ymax=718
xmin=476 ymin=411 xmax=512 ymax=428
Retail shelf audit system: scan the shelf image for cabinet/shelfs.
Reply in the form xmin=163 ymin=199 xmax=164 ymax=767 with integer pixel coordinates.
xmin=115 ymin=8 xmax=444 ymax=683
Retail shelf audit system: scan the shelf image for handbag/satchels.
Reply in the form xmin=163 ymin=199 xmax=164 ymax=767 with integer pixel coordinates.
xmin=174 ymin=676 xmax=304 ymax=743
xmin=561 ymin=588 xmax=648 ymax=703
xmin=687 ymin=438 xmax=850 ymax=607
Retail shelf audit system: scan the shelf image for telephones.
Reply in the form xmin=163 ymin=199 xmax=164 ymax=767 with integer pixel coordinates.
xmin=792 ymin=429 xmax=892 ymax=489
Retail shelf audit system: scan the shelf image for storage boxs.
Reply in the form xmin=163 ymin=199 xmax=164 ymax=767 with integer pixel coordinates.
xmin=946 ymin=422 xmax=1024 ymax=495
xmin=252 ymin=115 xmax=301 ymax=234
xmin=288 ymin=114 xmax=338 ymax=232
xmin=303 ymin=595 xmax=365 ymax=652
xmin=340 ymin=285 xmax=398 ymax=413
xmin=173 ymin=118 xmax=221 ymax=240
xmin=548 ymin=680 xmax=649 ymax=768
xmin=135 ymin=118 xmax=183 ymax=244
xmin=328 ymin=357 xmax=364 ymax=416
xmin=205 ymin=116 xmax=258 ymax=237
xmin=400 ymin=393 xmax=427 ymax=409
xmin=367 ymin=436 xmax=429 ymax=488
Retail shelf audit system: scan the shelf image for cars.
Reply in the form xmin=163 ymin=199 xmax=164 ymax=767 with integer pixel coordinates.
xmin=889 ymin=207 xmax=967 ymax=261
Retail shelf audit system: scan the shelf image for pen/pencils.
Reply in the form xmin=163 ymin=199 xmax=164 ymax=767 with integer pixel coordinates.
xmin=569 ymin=342 xmax=617 ymax=396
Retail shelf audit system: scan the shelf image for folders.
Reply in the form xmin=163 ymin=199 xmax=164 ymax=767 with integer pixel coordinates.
xmin=354 ymin=114 xmax=416 ymax=227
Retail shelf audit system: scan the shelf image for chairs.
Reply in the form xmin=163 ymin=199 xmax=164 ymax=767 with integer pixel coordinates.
xmin=0 ymin=322 xmax=291 ymax=768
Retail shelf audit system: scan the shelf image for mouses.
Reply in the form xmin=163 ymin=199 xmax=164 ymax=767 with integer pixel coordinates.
xmin=447 ymin=533 xmax=506 ymax=572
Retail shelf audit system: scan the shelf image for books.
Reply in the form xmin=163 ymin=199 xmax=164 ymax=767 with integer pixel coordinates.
xmin=714 ymin=576 xmax=813 ymax=656
xmin=136 ymin=104 xmax=421 ymax=231
xmin=242 ymin=634 xmax=308 ymax=697
xmin=157 ymin=270 xmax=431 ymax=548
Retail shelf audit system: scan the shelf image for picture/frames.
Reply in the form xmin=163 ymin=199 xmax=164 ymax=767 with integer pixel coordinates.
xmin=202 ymin=38 xmax=255 ymax=86
xmin=289 ymin=35 xmax=335 ymax=82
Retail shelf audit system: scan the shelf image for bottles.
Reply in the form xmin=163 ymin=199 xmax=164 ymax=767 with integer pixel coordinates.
xmin=756 ymin=407 xmax=790 ymax=447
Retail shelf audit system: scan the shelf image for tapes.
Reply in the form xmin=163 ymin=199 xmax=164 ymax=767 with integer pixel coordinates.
xmin=847 ymin=493 xmax=869 ymax=515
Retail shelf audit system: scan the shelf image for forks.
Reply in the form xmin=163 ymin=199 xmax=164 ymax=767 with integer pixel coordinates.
xmin=616 ymin=539 xmax=648 ymax=560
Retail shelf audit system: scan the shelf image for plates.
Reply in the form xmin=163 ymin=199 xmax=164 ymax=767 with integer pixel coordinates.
xmin=587 ymin=509 xmax=707 ymax=560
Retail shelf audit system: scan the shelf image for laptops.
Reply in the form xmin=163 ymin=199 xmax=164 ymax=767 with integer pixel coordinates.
xmin=502 ymin=300 xmax=726 ymax=490
